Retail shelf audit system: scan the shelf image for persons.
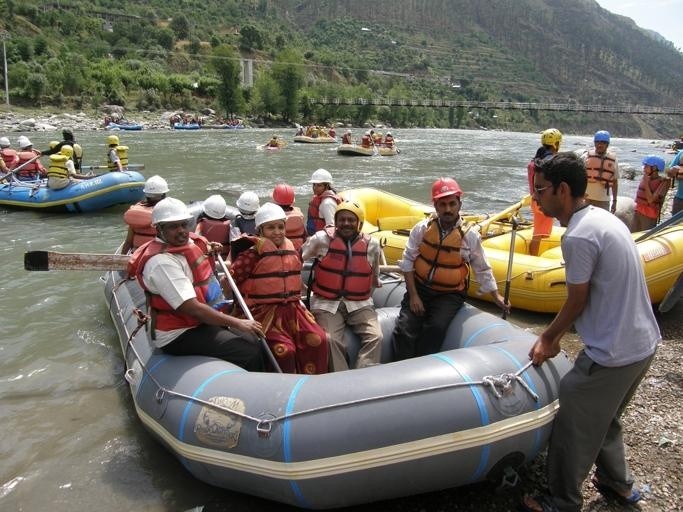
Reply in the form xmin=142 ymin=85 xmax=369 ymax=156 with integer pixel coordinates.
xmin=391 ymin=178 xmax=511 ymax=361
xmin=271 ymin=183 xmax=306 ymax=258
xmin=103 ymin=112 xmax=394 ymax=148
xmin=630 ymin=155 xmax=670 ymax=234
xmin=666 ymin=150 xmax=683 ymax=216
xmin=231 ymin=190 xmax=260 ymax=237
xmin=523 ymin=151 xmax=662 ymax=510
xmin=305 ymin=168 xmax=341 ymax=239
xmin=107 ymin=136 xmax=128 ymax=172
xmin=119 ymin=174 xmax=170 ymax=256
xmin=139 ymin=198 xmax=267 ymax=372
xmin=298 ymin=200 xmax=383 ymax=373
xmin=0 ymin=128 xmax=84 ymax=192
xmin=195 ymin=194 xmax=231 ymax=260
xmin=527 ymin=128 xmax=562 ymax=256
xmin=218 ymin=203 xmax=328 ymax=375
xmin=577 ymin=130 xmax=619 ymax=213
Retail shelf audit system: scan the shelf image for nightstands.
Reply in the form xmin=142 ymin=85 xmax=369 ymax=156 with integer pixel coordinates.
xmin=473 ymin=193 xmax=531 ymax=232
xmin=369 ymin=135 xmax=379 ymax=155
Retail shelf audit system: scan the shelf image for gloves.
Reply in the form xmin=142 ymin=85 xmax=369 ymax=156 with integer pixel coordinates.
xmin=99 ymin=240 xmax=573 ymax=510
xmin=209 ymin=123 xmax=244 ymax=128
xmin=337 ymin=141 xmax=397 ymax=157
xmin=292 ymin=135 xmax=337 ymax=144
xmin=107 ymin=121 xmax=143 ymax=130
xmin=332 ymin=184 xmax=683 ymax=314
xmin=0 ymin=168 xmax=147 ymax=217
xmin=264 ymin=138 xmax=289 ymax=149
xmin=174 ymin=121 xmax=199 ymax=130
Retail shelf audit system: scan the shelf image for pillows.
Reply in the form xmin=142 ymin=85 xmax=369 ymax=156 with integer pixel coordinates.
xmin=540 ymin=128 xmax=561 ymax=150
xmin=49 ymin=140 xmax=60 ymax=150
xmin=141 ymin=174 xmax=170 ymax=196
xmin=150 ymin=197 xmax=195 ymax=225
xmin=307 ymin=168 xmax=332 ymax=186
xmin=0 ymin=135 xmax=32 ymax=150
xmin=641 ymin=156 xmax=665 ymax=173
xmin=592 ymin=130 xmax=610 ymax=145
xmin=234 ymin=183 xmax=294 ymax=230
xmin=199 ymin=194 xmax=228 ymax=219
xmin=59 ymin=145 xmax=73 ymax=158
xmin=333 ymin=200 xmax=364 ymax=233
xmin=106 ymin=135 xmax=119 ymax=145
xmin=431 ymin=176 xmax=463 ymax=201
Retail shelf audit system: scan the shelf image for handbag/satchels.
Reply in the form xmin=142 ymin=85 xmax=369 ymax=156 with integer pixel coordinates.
xmin=612 ymin=200 xmax=616 ymax=201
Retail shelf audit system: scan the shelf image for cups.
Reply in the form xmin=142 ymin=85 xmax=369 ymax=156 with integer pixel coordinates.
xmin=532 ymin=182 xmax=553 ymax=195
